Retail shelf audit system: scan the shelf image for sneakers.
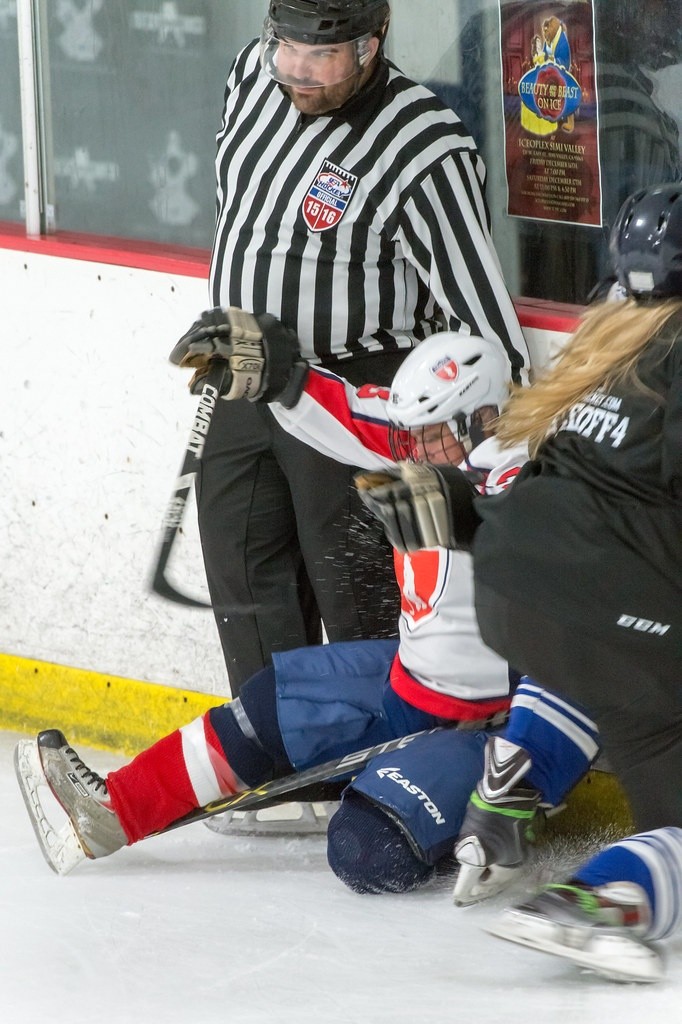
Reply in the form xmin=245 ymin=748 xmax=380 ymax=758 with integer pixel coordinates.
xmin=454 ymin=731 xmax=564 ymax=906
xmin=205 ymin=794 xmax=345 ymax=835
xmin=486 ymin=881 xmax=662 ymax=984
xmin=12 ymin=728 xmax=131 ymax=876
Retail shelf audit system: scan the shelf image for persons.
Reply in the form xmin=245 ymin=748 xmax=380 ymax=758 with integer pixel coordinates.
xmin=423 ymin=0 xmax=682 ymax=308
xmin=448 ymin=185 xmax=682 ymax=986
xmin=12 ymin=309 xmax=533 ymax=894
xmin=192 ymin=0 xmax=534 ymax=700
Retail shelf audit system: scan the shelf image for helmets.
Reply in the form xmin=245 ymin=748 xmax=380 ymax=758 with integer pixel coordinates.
xmin=384 ymin=332 xmax=514 ymax=454
xmin=609 ymin=180 xmax=682 ymax=304
xmin=267 ymin=0 xmax=390 ymax=46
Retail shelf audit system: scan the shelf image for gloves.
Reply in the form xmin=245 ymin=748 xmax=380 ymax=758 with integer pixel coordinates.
xmin=355 ymin=459 xmax=483 ymax=554
xmin=171 ymin=308 xmax=309 ymax=410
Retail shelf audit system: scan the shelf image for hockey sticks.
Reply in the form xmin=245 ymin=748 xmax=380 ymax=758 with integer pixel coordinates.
xmin=148 ymin=356 xmax=274 ymax=617
xmin=131 ymin=711 xmax=516 ymax=844
